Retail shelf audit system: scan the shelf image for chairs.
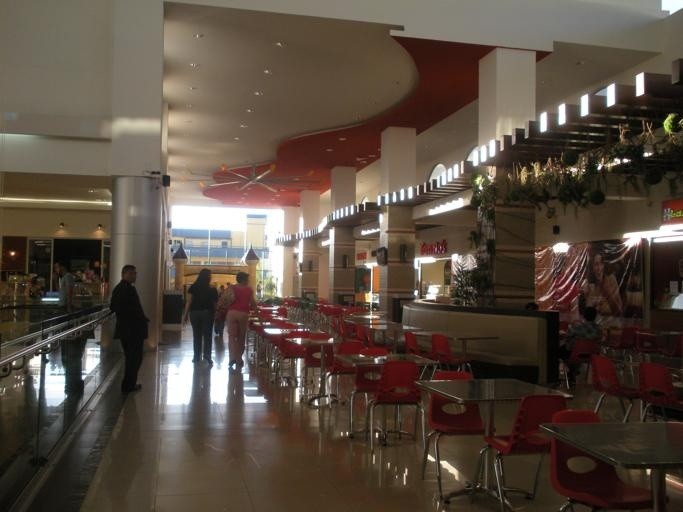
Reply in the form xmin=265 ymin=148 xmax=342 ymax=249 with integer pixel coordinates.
xmin=424 ymin=370 xmax=486 ymax=506
xmin=247 ymin=299 xmax=682 ymax=410
xmin=362 ymin=359 xmax=420 ymax=443
xmin=592 ymin=354 xmax=648 ymax=423
xmin=482 ymin=392 xmax=568 ymax=510
xmin=549 ymin=410 xmax=669 ymax=511
xmin=637 ymin=361 xmax=683 ymax=421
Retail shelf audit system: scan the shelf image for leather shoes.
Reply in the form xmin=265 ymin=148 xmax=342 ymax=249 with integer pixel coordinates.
xmin=122 ymin=384 xmax=142 ymax=392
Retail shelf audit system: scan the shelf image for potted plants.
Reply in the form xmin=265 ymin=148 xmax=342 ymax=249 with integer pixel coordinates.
xmin=661 ymin=114 xmax=682 ymax=148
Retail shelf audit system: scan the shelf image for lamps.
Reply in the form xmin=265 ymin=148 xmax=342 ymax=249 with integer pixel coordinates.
xmin=198 ymin=164 xmax=302 ymax=194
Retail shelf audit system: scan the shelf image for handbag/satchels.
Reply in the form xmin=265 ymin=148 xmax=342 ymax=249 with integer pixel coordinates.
xmin=218 ymin=288 xmax=235 ymax=310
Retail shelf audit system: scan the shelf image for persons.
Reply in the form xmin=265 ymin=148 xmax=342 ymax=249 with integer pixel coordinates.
xmin=581 ymin=253 xmax=623 ymax=314
xmin=523 ymin=302 xmax=539 ymax=310
xmin=182 ymin=268 xmax=263 ymax=367
xmin=52 ymin=261 xmax=73 ymax=313
xmin=561 ymin=306 xmax=603 ymax=361
xmin=29 ymin=277 xmax=46 ymax=296
xmin=111 ymin=265 xmax=150 ymax=393
